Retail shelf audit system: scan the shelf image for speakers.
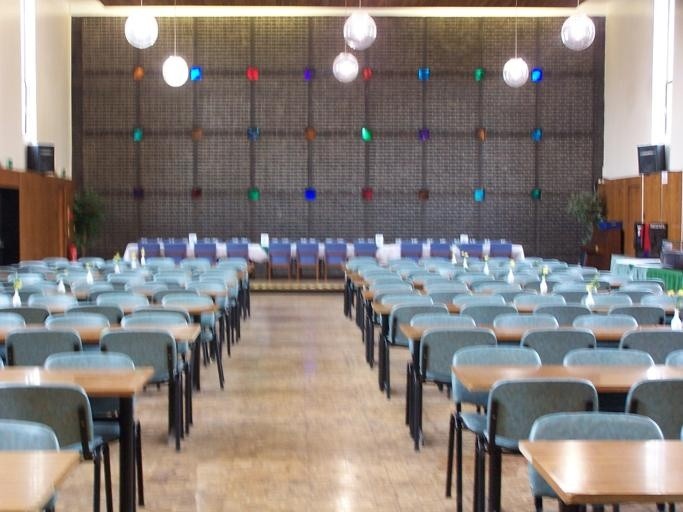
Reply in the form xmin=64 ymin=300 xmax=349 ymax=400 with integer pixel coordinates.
xmin=638 ymin=146 xmax=666 ymax=174
xmin=28 ymin=145 xmax=55 ymax=177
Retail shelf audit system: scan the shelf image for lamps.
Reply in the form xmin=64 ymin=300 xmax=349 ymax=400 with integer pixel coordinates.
xmin=501 ymin=0 xmax=595 ymax=90
xmin=331 ymin=0 xmax=376 ymax=85
xmin=123 ymin=0 xmax=190 ymax=88
